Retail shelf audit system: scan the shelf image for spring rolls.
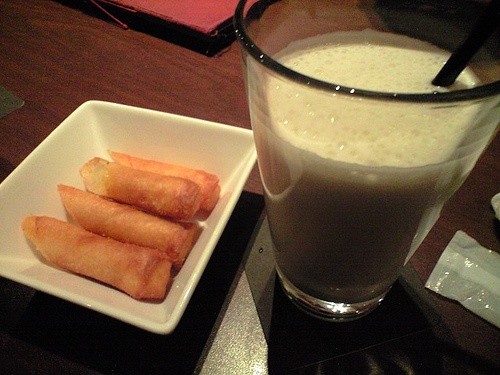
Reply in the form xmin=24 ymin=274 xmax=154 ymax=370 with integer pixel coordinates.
xmin=21 ymin=148 xmax=221 ymax=301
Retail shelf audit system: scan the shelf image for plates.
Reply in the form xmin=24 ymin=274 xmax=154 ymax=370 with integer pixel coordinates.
xmin=0 ymin=101 xmax=257 ymax=334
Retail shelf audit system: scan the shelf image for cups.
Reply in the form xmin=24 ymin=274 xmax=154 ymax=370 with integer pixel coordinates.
xmin=234 ymin=0 xmax=500 ymax=322
